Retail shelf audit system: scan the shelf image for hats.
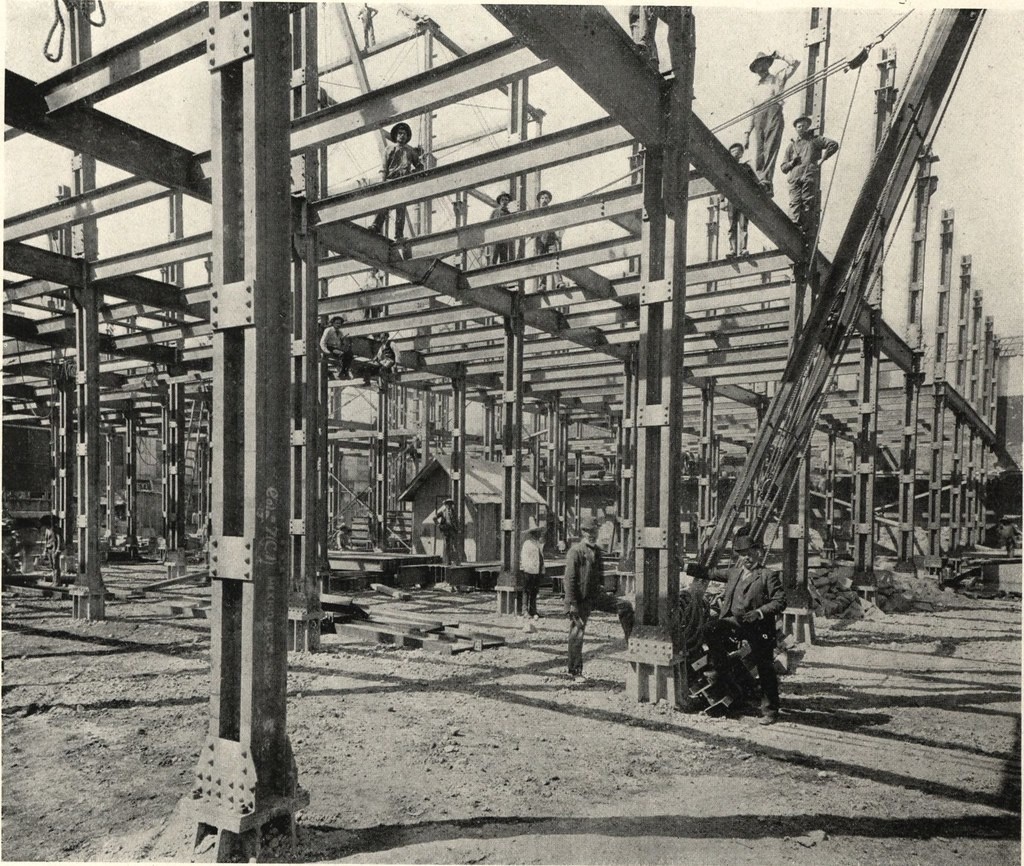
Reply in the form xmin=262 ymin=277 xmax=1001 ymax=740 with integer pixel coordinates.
xmin=497 ymin=191 xmax=513 ymax=205
xmin=537 ymin=190 xmax=552 ymax=204
xmin=390 ymin=122 xmax=411 ymax=144
xmin=442 ymin=498 xmax=454 ymax=505
xmin=580 ymin=516 xmax=602 ymax=531
xmin=749 ymin=51 xmax=774 ymax=73
xmin=728 ymin=142 xmax=744 ymax=155
xmin=330 ymin=317 xmax=344 ymax=324
xmin=521 ymin=516 xmax=546 ymax=534
xmin=793 ymin=113 xmax=812 ymax=130
xmin=734 ymin=535 xmax=761 ymax=552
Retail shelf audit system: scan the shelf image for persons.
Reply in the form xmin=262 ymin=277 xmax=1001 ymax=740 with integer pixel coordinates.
xmin=720 ymin=143 xmax=761 ymax=259
xmin=40 ymin=512 xmax=65 ymax=589
xmin=318 ymin=316 xmax=354 ymax=381
xmin=520 ymin=516 xmax=548 ymax=619
xmin=360 ymin=331 xmax=401 ymax=393
xmin=780 ymin=114 xmax=838 ymax=251
xmin=432 ymin=498 xmax=464 ymax=565
xmin=490 ymin=191 xmax=514 ymax=266
xmin=1000 ymin=519 xmax=1019 ymax=558
xmin=562 ymin=515 xmax=636 ymax=687
xmin=337 ymin=522 xmax=352 ymax=551
xmin=700 ymin=536 xmax=788 ymax=728
xmin=368 ymin=123 xmax=426 ymax=243
xmin=743 ymin=50 xmax=801 ymax=196
xmin=532 ymin=190 xmax=568 ymax=292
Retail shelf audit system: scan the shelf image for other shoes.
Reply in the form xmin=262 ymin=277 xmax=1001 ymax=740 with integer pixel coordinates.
xmin=573 ymin=674 xmax=585 ymax=683
xmin=759 ymin=715 xmax=779 ymax=726
xmin=537 ymin=285 xmax=546 ymax=292
xmin=557 ymin=283 xmax=566 ymax=289
xmin=740 ymin=250 xmax=749 ymax=256
xmin=703 ymin=669 xmax=731 ymax=678
xmin=726 ymin=252 xmax=737 ymax=258
xmin=396 ymin=237 xmax=408 ymax=243
xmin=368 ymin=225 xmax=379 ymax=232
